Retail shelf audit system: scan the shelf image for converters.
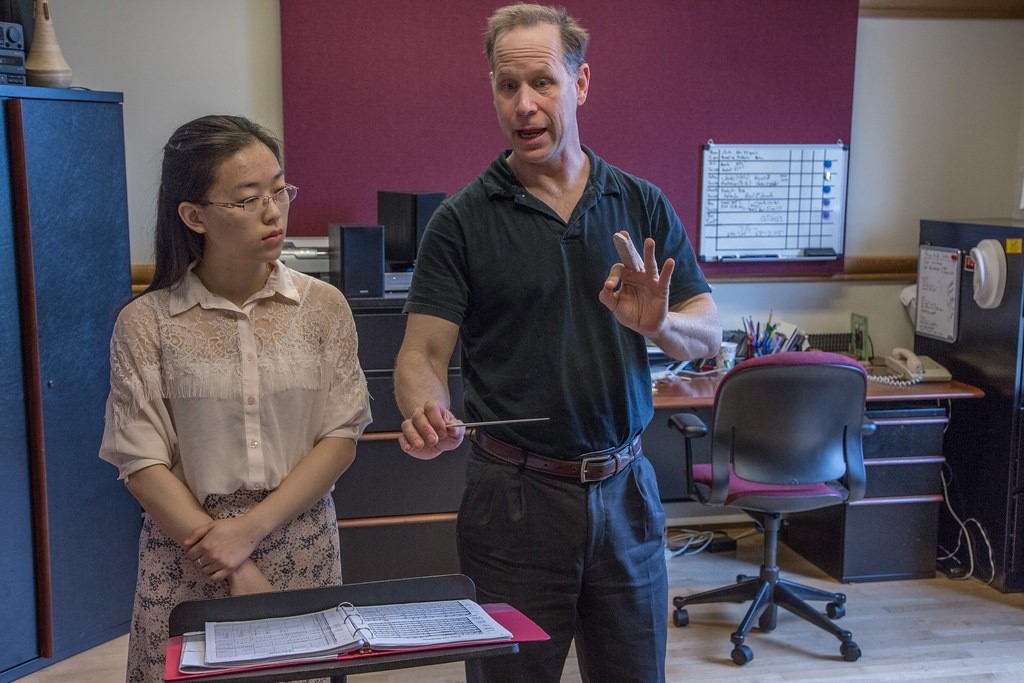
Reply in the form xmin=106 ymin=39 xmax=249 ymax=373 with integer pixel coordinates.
xmin=705 ymin=535 xmax=738 ymax=553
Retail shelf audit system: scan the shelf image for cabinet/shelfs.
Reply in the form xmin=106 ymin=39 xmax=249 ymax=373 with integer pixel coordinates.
xmin=0 ymin=86 xmax=145 ymax=683
xmin=331 ymin=298 xmax=474 ymax=585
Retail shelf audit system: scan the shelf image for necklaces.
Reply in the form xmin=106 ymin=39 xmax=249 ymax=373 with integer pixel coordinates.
xmin=261 ymin=262 xmax=270 ymax=290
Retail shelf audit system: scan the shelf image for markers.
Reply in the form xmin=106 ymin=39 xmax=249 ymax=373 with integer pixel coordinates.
xmin=721 ymin=255 xmax=744 ymax=259
xmin=745 ymin=254 xmax=779 ymax=258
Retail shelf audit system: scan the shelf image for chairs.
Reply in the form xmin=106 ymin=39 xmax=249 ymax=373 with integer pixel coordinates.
xmin=667 ymin=353 xmax=876 ymax=666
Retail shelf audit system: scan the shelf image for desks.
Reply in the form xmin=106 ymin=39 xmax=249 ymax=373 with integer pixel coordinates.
xmin=652 ymin=356 xmax=984 ymax=584
xmin=170 ymin=575 xmax=521 ymax=683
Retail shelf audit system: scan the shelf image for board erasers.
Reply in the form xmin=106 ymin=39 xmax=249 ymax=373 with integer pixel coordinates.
xmin=801 ymin=247 xmax=836 ymax=256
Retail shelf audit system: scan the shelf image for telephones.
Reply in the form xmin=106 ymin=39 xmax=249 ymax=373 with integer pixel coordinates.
xmin=886 ymin=347 xmax=952 ymax=382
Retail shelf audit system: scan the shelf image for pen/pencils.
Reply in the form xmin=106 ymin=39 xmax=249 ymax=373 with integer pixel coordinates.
xmin=763 ymin=313 xmax=772 ymax=341
xmin=741 ymin=315 xmax=759 ymax=345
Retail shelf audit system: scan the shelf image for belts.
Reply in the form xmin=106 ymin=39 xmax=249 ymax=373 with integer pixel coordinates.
xmin=473 ymin=429 xmax=642 ymax=483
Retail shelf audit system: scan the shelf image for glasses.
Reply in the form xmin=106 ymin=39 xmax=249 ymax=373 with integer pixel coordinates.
xmin=194 ymin=182 xmax=299 ymax=214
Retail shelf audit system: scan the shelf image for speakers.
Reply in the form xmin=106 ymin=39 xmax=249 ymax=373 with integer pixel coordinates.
xmin=326 ymin=191 xmax=447 ymax=300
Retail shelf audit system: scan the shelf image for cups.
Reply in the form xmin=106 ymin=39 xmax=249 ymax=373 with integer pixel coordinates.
xmin=750 ymin=344 xmax=765 ymax=359
xmin=715 ymin=342 xmax=738 ymax=375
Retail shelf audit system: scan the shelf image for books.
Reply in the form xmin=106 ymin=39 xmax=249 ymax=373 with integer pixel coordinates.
xmin=178 ymin=598 xmax=513 ymax=673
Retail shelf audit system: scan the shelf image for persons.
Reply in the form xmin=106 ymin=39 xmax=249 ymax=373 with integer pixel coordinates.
xmin=101 ymin=115 xmax=373 ymax=683
xmin=393 ymin=2 xmax=720 ymax=683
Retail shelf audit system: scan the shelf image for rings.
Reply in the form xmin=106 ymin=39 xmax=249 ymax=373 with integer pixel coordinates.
xmin=646 ymin=269 xmax=658 ymax=274
xmin=197 ymin=558 xmax=203 ymax=568
xmin=630 ymin=271 xmax=633 ymax=283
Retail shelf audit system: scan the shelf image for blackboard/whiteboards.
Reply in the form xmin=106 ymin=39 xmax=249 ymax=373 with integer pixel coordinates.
xmin=914 ymin=244 xmax=961 ymax=343
xmin=698 ymin=139 xmax=848 ymax=263
